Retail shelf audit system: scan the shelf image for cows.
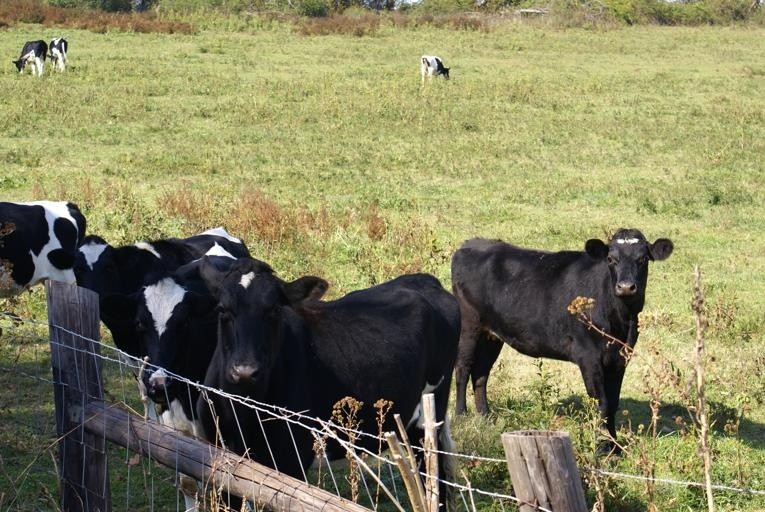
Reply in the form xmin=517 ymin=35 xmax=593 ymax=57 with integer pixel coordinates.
xmin=0 ymin=199 xmax=87 ymax=299
xmin=11 ymin=40 xmax=48 ymax=77
xmin=66 ymin=224 xmax=463 ymax=511
xmin=450 ymin=228 xmax=675 ymax=463
xmin=420 ymin=54 xmax=451 ymax=84
xmin=47 ymin=37 xmax=71 ymax=73
